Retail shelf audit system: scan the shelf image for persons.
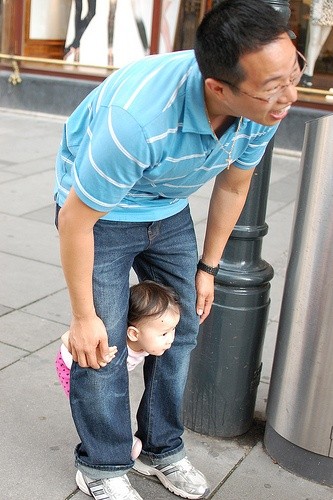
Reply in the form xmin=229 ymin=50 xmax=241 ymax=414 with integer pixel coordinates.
xmin=55 ymin=280 xmax=184 ymax=462
xmin=54 ymin=0 xmax=311 ymax=499
xmin=298 ymin=0 xmax=333 ymax=87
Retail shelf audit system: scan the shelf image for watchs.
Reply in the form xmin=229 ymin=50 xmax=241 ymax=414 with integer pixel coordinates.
xmin=197 ymin=260 xmax=221 ymax=277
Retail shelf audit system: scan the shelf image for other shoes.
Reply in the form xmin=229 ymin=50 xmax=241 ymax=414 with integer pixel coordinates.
xmin=130 ymin=435 xmax=142 ymax=461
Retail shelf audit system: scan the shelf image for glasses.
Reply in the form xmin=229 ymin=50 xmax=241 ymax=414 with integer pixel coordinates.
xmin=212 ymin=50 xmax=308 ymax=104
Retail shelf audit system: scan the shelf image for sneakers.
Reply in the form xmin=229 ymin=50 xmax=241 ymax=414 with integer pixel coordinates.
xmin=132 ymin=456 xmax=210 ymax=499
xmin=76 ymin=469 xmax=144 ymax=500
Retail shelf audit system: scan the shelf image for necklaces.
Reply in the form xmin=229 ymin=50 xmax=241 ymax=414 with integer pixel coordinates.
xmin=204 ymin=95 xmax=243 ymax=170
